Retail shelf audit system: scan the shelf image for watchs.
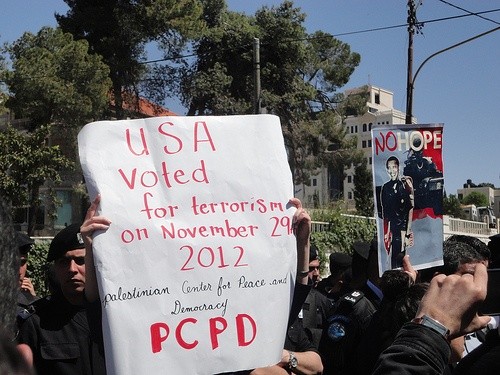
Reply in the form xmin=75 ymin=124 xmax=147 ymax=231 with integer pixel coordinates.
xmin=285 ymin=350 xmax=298 ymax=372
xmin=411 ymin=315 xmax=451 ymax=344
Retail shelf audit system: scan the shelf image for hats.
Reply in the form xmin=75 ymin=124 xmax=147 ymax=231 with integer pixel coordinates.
xmin=47 ymin=224 xmax=85 ymax=262
xmin=16 ymin=232 xmax=35 ymax=253
xmin=330 ymin=253 xmax=352 ymax=269
xmin=309 ymin=246 xmax=320 ymax=264
xmin=371 ymin=237 xmax=378 ymax=252
xmin=488 ymin=234 xmax=500 ymax=241
xmin=350 ymin=242 xmax=371 ymax=260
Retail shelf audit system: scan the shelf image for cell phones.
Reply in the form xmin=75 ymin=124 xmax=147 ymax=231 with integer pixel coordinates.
xmin=477 ymin=269 xmax=500 ymax=316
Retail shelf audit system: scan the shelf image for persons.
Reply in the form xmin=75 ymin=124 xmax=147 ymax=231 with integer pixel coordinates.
xmin=0 ymin=195 xmax=500 ymax=375
xmin=403 ymin=148 xmax=437 ymax=189
xmin=380 ymin=156 xmax=414 ymax=269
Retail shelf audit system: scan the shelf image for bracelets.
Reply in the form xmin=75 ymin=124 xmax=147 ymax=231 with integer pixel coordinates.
xmin=297 ymin=271 xmax=310 ymax=277
